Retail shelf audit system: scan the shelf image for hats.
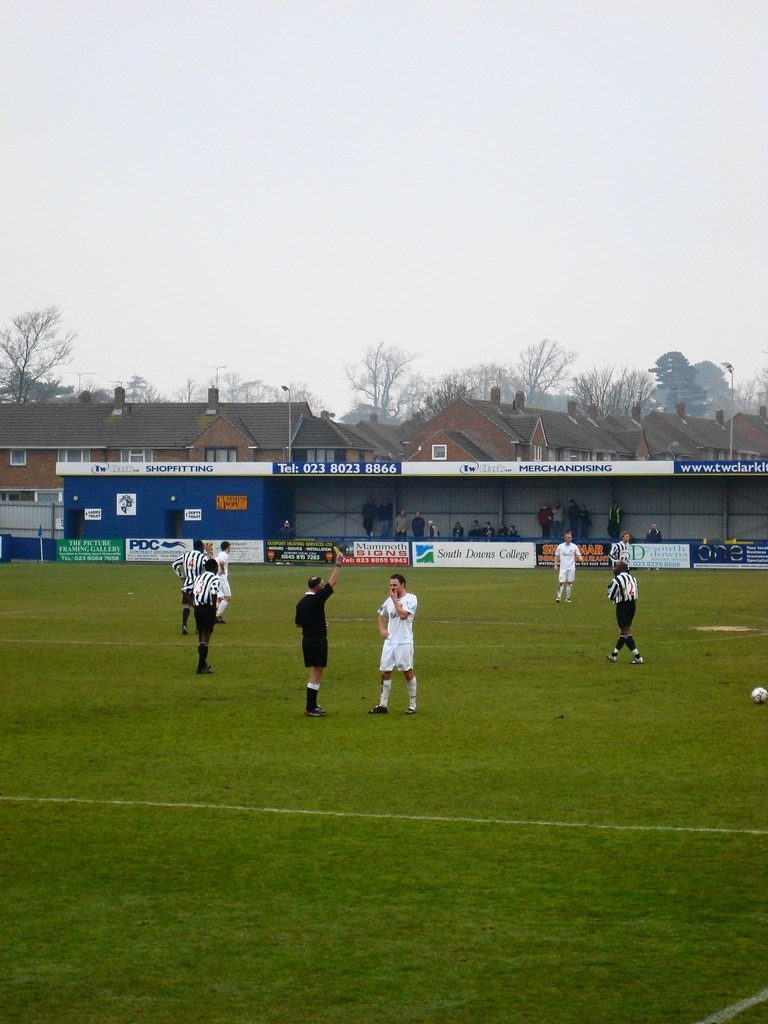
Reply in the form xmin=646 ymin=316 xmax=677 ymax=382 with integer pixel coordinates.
xmin=427 ymin=520 xmax=433 ymax=524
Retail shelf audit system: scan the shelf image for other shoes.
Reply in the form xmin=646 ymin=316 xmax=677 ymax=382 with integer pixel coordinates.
xmin=648 ymin=568 xmax=653 ymax=571
xmin=215 ymin=616 xmax=226 ymax=623
xmin=196 ymin=628 xmax=199 ymax=633
xmin=656 ymin=568 xmax=660 ymax=571
xmin=566 ymin=598 xmax=571 ymax=602
xmin=196 ymin=665 xmax=213 ymax=673
xmin=556 ymin=597 xmax=561 ymax=603
xmin=182 ymin=626 xmax=187 ymax=634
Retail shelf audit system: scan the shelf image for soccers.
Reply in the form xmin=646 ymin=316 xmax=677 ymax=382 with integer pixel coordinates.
xmin=750 ymin=687 xmax=768 ymax=704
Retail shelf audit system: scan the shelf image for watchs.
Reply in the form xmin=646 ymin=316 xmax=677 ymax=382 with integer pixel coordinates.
xmin=335 ymin=564 xmax=341 ymax=567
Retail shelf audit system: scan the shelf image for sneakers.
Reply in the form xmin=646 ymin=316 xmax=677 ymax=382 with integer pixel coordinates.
xmin=368 ymin=705 xmax=388 ymax=713
xmin=630 ymin=657 xmax=643 ymax=664
xmin=606 ymin=655 xmax=617 ymax=662
xmin=406 ymin=707 xmax=416 ymax=714
xmin=305 ymin=705 xmax=328 ymax=716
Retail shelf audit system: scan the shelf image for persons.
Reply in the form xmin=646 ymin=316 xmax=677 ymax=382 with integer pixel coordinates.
xmin=470 ymin=520 xmax=482 ymax=542
xmin=508 ymin=525 xmax=521 ymax=542
xmin=453 ymin=521 xmax=464 ymax=538
xmin=377 ymin=496 xmax=393 ymax=538
xmin=412 ymin=511 xmax=425 ymax=541
xmin=646 ymin=523 xmax=662 ymax=544
xmin=568 ymin=500 xmax=580 ymax=540
xmin=609 ymin=531 xmax=632 ymax=577
xmin=214 ymin=542 xmax=232 ymax=624
xmin=579 ymin=504 xmax=588 ymax=539
xmin=367 ymin=572 xmax=417 ymax=714
xmin=361 ymin=497 xmax=377 ymax=541
xmin=192 ymin=559 xmax=220 ymax=674
xmin=607 ymin=500 xmax=623 ymax=539
xmin=482 ymin=522 xmax=495 ymax=542
xmin=427 ymin=520 xmax=440 ymax=542
xmin=537 ymin=502 xmax=554 ymax=539
xmin=295 ymin=552 xmax=344 ymax=718
xmin=172 ymin=540 xmax=209 ymax=634
xmin=554 ymin=533 xmax=583 ymax=603
xmin=606 ymin=560 xmax=643 ymax=664
xmin=552 ymin=500 xmax=564 ymax=539
xmin=394 ymin=509 xmax=410 ymax=542
xmin=498 ymin=523 xmax=508 ymax=536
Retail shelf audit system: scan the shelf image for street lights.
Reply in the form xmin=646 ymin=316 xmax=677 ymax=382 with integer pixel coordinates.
xmin=282 ymin=386 xmax=291 ymax=462
xmin=721 ymin=362 xmax=734 ymax=460
